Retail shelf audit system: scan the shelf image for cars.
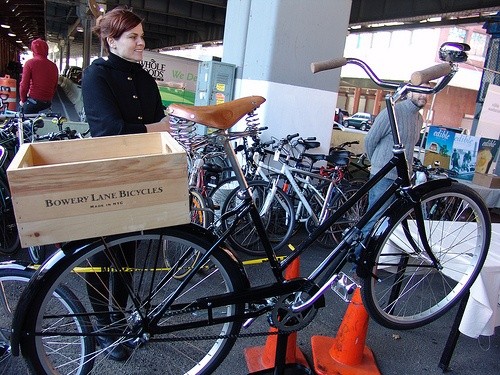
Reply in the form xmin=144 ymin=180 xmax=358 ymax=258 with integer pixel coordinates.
xmin=343 ymin=112 xmax=376 ymax=130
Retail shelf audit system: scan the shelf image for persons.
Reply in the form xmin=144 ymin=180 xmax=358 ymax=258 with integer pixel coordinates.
xmin=16 ymin=37 xmax=59 ymax=115
xmin=81 ymin=6 xmax=171 ymax=363
xmin=353 ymin=78 xmax=431 ymax=283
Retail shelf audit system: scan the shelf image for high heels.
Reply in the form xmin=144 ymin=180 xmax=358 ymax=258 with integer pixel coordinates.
xmin=92 ymin=314 xmax=143 ymax=361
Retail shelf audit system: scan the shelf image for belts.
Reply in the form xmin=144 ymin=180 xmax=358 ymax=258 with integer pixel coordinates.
xmin=27 ymin=98 xmax=52 ymax=104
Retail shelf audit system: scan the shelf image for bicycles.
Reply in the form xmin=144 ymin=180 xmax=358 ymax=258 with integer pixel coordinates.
xmin=0 ymin=58 xmax=491 ymax=375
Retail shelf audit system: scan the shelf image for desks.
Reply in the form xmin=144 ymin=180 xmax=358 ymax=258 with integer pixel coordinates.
xmin=376 ymin=219 xmax=500 ymax=370
xmin=431 ymin=175 xmax=500 ymax=208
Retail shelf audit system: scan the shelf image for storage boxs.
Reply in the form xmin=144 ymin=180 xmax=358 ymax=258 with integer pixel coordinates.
xmin=473 ymin=173 xmax=500 ymax=188
xmin=6 ymin=131 xmax=191 ymax=248
